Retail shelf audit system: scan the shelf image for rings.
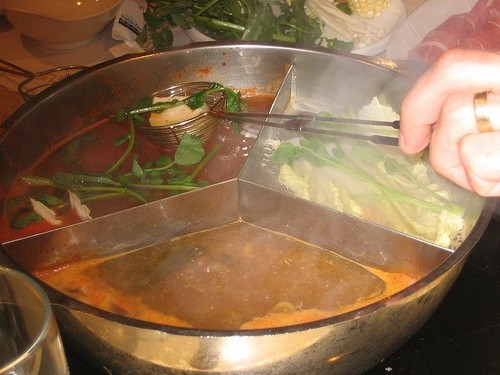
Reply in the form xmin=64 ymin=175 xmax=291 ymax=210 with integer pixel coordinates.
xmin=473 ymin=87 xmax=495 ymax=133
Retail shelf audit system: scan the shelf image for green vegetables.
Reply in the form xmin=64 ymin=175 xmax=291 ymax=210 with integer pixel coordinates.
xmin=136 ymin=0 xmax=353 ymax=55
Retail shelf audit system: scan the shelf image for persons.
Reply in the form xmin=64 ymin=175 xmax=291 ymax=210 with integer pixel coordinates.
xmin=398 ymin=49 xmax=500 ymax=197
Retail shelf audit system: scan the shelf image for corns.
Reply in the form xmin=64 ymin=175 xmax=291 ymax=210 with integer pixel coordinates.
xmin=347 ymin=0 xmax=391 ymax=18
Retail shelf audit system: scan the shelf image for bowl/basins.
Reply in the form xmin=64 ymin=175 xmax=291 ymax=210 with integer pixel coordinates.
xmin=0 ymin=0 xmax=124 ymax=51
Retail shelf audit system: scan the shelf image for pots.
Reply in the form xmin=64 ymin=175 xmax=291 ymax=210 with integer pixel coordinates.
xmin=0 ymin=41 xmax=500 ymax=375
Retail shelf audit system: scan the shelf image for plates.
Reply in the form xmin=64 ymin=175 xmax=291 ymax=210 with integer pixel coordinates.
xmin=385 ymin=0 xmax=500 ymax=57
xmin=181 ymin=0 xmax=407 ymax=56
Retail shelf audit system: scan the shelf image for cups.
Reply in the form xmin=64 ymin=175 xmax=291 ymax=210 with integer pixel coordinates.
xmin=0 ymin=268 xmax=70 ymax=375
